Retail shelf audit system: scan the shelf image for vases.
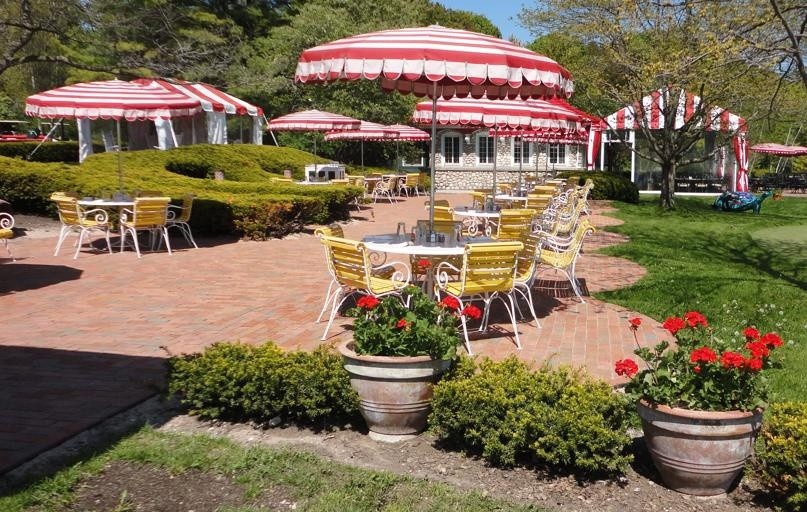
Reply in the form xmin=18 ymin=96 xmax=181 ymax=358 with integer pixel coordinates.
xmin=631 ymin=389 xmax=766 ymax=497
xmin=339 ymin=337 xmax=456 ymax=443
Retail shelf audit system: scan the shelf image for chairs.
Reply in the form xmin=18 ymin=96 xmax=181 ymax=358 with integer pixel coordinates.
xmin=330 ymin=172 xmax=427 ymax=205
xmin=0 ymin=211 xmax=19 ymax=266
xmin=424 ymin=200 xmax=521 ymax=219
xmin=469 ymin=182 xmax=516 ymax=200
xmin=516 ymin=176 xmax=596 ymax=304
xmin=314 ymin=220 xmax=547 ymax=355
xmin=50 ymin=186 xmax=198 ymax=261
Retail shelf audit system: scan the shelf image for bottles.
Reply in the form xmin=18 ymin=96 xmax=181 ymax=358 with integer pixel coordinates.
xmin=411 ymin=230 xmax=445 ymax=243
xmin=481 ymin=201 xmax=502 ymax=211
xmin=510 ymin=190 xmax=528 ymax=197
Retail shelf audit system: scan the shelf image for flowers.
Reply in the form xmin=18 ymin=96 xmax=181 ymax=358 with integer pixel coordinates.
xmin=614 ymin=309 xmax=787 ymax=401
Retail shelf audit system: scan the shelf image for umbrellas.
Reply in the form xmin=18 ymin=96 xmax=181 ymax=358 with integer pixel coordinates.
xmin=749 ymin=143 xmax=807 ymax=175
xmin=25 ymin=76 xmax=202 ymax=242
xmin=296 ymin=21 xmax=575 ymax=300
